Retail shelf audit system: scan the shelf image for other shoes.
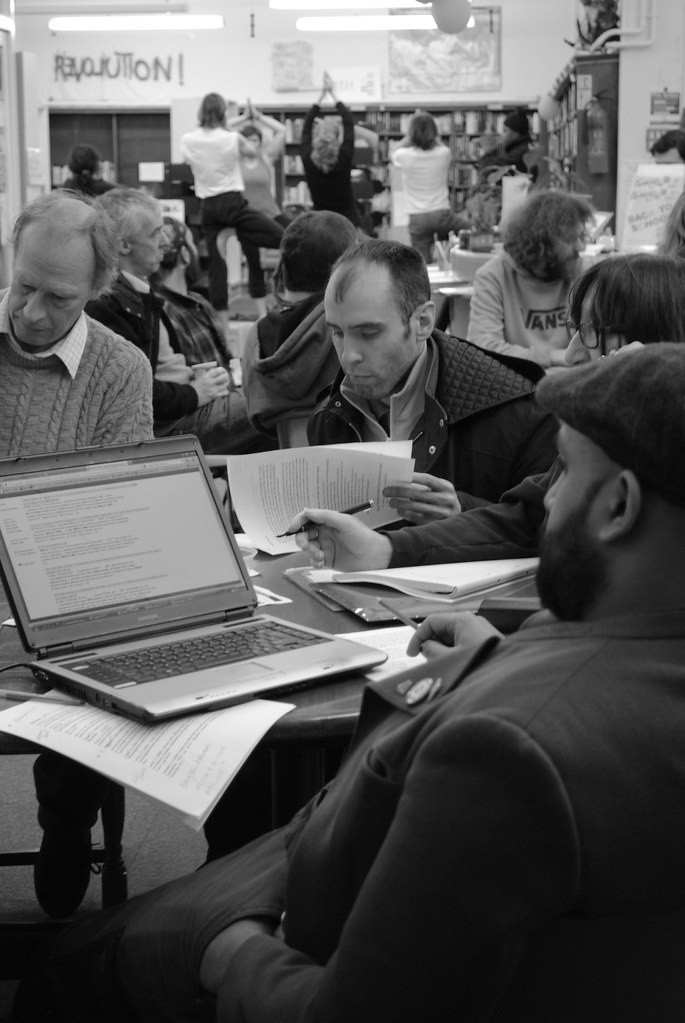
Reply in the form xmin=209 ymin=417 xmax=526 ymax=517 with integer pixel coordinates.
xmin=35 ymin=822 xmax=101 ymax=918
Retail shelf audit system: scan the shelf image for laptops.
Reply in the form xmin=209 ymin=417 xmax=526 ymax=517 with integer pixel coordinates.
xmin=0 ymin=434 xmax=388 ymax=728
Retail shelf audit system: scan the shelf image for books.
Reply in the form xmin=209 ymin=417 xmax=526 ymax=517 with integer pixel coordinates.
xmin=364 ymin=112 xmax=414 ymax=181
xmin=282 ymin=557 xmax=540 ymax=622
xmin=434 ymin=111 xmax=540 ymax=211
xmin=547 ymin=84 xmax=576 ymax=155
xmin=282 ymin=119 xmax=313 ymax=206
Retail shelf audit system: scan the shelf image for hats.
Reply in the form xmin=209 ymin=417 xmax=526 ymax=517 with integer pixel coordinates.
xmin=163 ymin=216 xmax=185 ymax=263
xmin=533 ymin=337 xmax=684 ymax=510
xmin=504 ymin=112 xmax=530 ymax=137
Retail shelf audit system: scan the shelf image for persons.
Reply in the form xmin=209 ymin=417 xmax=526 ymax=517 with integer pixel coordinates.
xmin=466 ymin=192 xmax=596 ymax=368
xmin=307 ymin=241 xmax=561 ymax=526
xmin=242 ymin=210 xmax=358 ymax=449
xmin=649 ymin=129 xmax=685 ymax=252
xmin=288 ymin=252 xmax=685 ymax=573
xmin=150 ymin=217 xmax=233 ymax=366
xmin=388 ymin=108 xmax=472 ymax=265
xmin=84 ymin=187 xmax=259 ymax=453
xmin=63 ymin=145 xmax=117 ymax=198
xmin=300 ymin=70 xmax=361 ymax=229
xmin=226 ymin=97 xmax=294 ymax=321
xmin=11 ymin=343 xmax=685 ymax=1023
xmin=478 ymin=113 xmax=539 ymax=225
xmin=0 ymin=187 xmax=155 ymax=919
xmin=181 ymin=93 xmax=285 ymax=312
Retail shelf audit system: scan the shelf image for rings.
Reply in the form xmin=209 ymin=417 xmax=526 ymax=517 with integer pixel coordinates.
xmin=315 ymin=529 xmax=319 ymax=538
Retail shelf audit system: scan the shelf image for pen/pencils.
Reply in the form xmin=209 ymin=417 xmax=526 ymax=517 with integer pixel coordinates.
xmin=377 ymin=597 xmax=419 ymax=629
xmin=0 ymin=690 xmax=86 ymax=707
xmin=277 ymin=500 xmax=374 ymax=538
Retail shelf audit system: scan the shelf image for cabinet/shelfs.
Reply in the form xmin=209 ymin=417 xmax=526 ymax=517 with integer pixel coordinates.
xmin=237 ymin=51 xmax=619 ymax=232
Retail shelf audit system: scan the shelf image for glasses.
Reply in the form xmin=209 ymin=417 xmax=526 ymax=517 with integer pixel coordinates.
xmin=566 ymin=318 xmax=627 ymax=349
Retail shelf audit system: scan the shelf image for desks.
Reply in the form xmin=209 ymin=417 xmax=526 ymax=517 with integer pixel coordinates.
xmin=0 ymin=528 xmax=364 ymax=913
